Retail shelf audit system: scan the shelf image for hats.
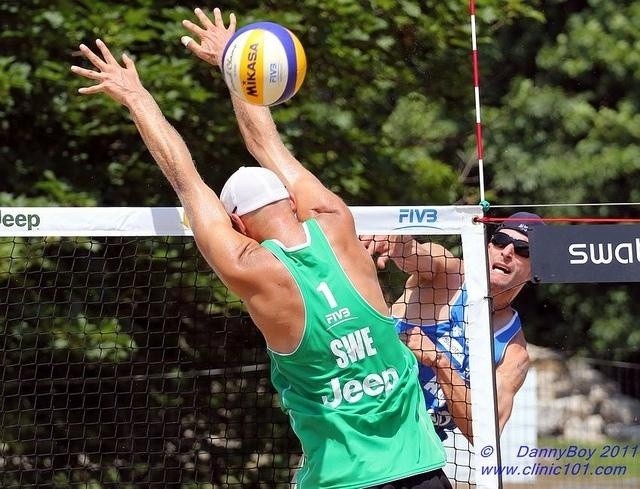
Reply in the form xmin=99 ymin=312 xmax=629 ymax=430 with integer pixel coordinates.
xmin=496 ymin=211 xmax=546 ymax=237
xmin=220 ymin=166 xmax=290 ymax=217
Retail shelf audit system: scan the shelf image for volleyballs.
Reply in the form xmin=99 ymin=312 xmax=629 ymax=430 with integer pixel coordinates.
xmin=222 ymin=21 xmax=308 ymax=107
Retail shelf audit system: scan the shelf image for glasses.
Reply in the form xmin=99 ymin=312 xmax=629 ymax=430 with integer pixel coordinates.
xmin=490 ymin=232 xmax=529 ymax=258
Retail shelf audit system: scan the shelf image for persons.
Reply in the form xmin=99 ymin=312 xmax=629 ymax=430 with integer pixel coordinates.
xmin=356 ymin=210 xmax=548 ymax=449
xmin=69 ymin=6 xmax=452 ymax=489
